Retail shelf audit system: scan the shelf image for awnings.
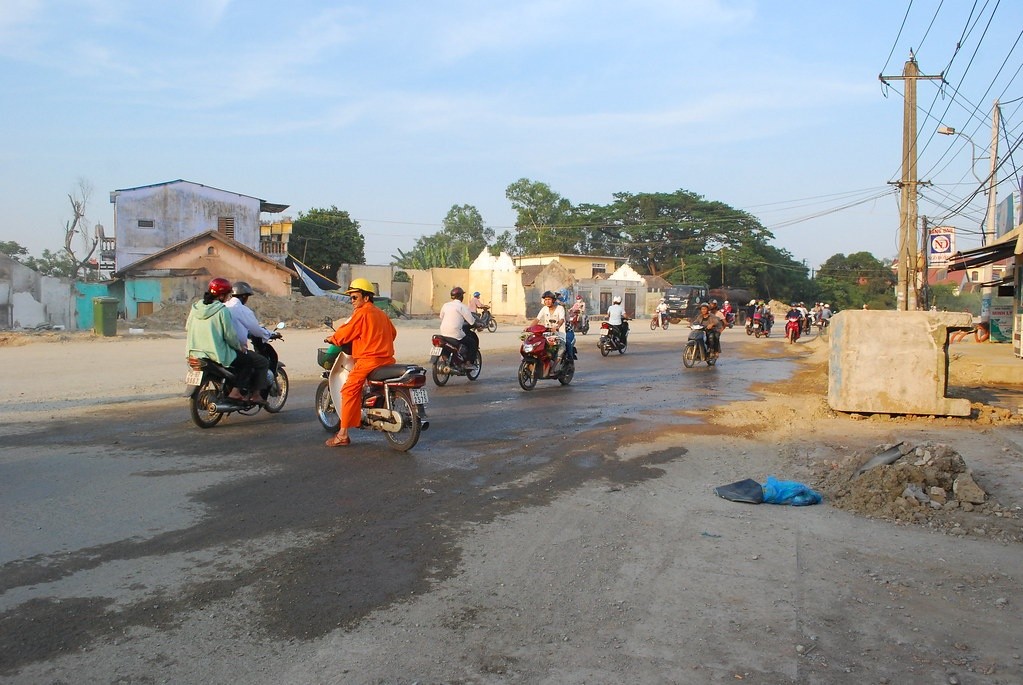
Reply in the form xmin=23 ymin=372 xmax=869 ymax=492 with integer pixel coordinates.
xmin=980 ymin=275 xmax=1014 ymax=287
xmin=946 ymin=240 xmax=1016 ymax=274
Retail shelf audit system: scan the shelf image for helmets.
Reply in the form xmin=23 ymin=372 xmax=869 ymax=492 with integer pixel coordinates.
xmin=614 ymin=296 xmax=623 ymax=304
xmin=555 ymin=289 xmax=569 ymax=300
xmin=749 ymin=299 xmax=764 ymax=306
xmin=230 ymin=282 xmax=255 ymax=296
xmin=660 ymin=298 xmax=666 ymax=302
xmin=450 ymin=287 xmax=467 ymax=299
xmin=576 ymin=295 xmax=583 ymax=300
xmin=724 ymin=301 xmax=729 ymax=305
xmin=208 ymin=278 xmax=234 ymax=297
xmin=791 ymin=302 xmax=804 ymax=308
xmin=699 ymin=303 xmax=709 ymax=309
xmin=342 ymin=278 xmax=376 ymax=296
xmin=815 ymin=302 xmax=830 ymax=308
xmin=709 ymin=299 xmax=718 ymax=306
xmin=541 ymin=290 xmax=556 ymax=301
xmin=473 ymin=292 xmax=480 ymax=298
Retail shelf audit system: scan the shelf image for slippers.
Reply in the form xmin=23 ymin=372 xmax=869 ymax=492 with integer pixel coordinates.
xmin=248 ymin=395 xmax=270 ymax=407
xmin=227 ymin=394 xmax=249 ymax=404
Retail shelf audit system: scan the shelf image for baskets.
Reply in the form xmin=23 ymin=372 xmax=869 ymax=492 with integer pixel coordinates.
xmin=317 ymin=348 xmax=339 ymax=370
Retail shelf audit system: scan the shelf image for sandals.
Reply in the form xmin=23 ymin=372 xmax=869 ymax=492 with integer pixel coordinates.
xmin=325 ymin=433 xmax=351 ymax=446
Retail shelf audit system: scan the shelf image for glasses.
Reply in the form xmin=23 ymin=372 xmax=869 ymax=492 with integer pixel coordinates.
xmin=349 ymin=296 xmax=357 ymax=303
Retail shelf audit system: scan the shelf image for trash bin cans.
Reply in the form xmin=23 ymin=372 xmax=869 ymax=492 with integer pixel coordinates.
xmin=373 ymin=296 xmax=388 ymax=309
xmin=93 ymin=296 xmax=120 ymax=337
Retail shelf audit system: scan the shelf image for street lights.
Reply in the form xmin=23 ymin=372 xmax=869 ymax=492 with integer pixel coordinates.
xmin=937 ymin=127 xmax=997 ymax=342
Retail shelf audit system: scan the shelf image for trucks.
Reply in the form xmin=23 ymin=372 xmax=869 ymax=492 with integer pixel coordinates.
xmin=664 ymin=285 xmax=749 ymax=326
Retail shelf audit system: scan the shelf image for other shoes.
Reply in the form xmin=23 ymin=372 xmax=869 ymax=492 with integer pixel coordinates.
xmin=784 ymin=334 xmax=788 ymax=338
xmin=764 ymin=330 xmax=769 ymax=335
xmin=620 ymin=340 xmax=627 ymax=347
xmin=465 ymin=360 xmax=478 ymax=371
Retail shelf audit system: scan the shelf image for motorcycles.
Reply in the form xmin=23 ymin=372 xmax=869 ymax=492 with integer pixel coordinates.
xmin=597 ymin=316 xmax=632 ymax=357
xmin=753 ymin=313 xmax=775 ymax=338
xmin=809 ymin=311 xmax=820 ymax=326
xmin=719 ymin=308 xmax=736 ymax=329
xmin=682 ymin=322 xmax=717 ymax=368
xmin=817 ymin=311 xmax=837 ymax=331
xmin=800 ymin=313 xmax=812 ymax=335
xmin=429 ymin=322 xmax=483 ymax=387
xmin=650 ymin=309 xmax=669 ymax=330
xmin=471 ymin=300 xmax=498 ymax=333
xmin=569 ymin=305 xmax=589 ymax=335
xmin=183 ymin=322 xmax=289 ymax=429
xmin=518 ymin=324 xmax=578 ymax=391
xmin=315 ymin=316 xmax=430 ymax=452
xmin=745 ymin=317 xmax=755 ymax=336
xmin=785 ymin=315 xmax=802 ymax=345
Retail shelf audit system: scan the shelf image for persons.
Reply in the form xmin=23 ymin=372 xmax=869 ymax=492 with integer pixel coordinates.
xmin=687 ymin=302 xmax=719 ymax=358
xmin=707 ymin=299 xmax=727 ymax=359
xmin=568 ymin=296 xmax=586 ymax=330
xmin=555 ymin=292 xmax=574 ymax=372
xmin=811 ymin=301 xmax=833 ymax=327
xmin=439 ymin=287 xmax=482 ymax=369
xmin=784 ymin=302 xmax=809 ymax=338
xmin=222 ymin=282 xmax=282 ymax=405
xmin=863 ymin=304 xmax=868 ymax=310
xmin=929 ymin=306 xmax=969 ymax=312
xmin=747 ymin=298 xmax=773 ymax=334
xmin=607 ymin=295 xmax=631 ymax=346
xmin=724 ymin=300 xmax=732 ymax=317
xmin=324 ymin=278 xmax=397 ymax=446
xmin=468 ymin=292 xmax=490 ymax=331
xmin=522 ymin=291 xmax=566 ymax=376
xmin=656 ymin=298 xmax=667 ymax=327
xmin=185 ymin=279 xmax=253 ymax=402
